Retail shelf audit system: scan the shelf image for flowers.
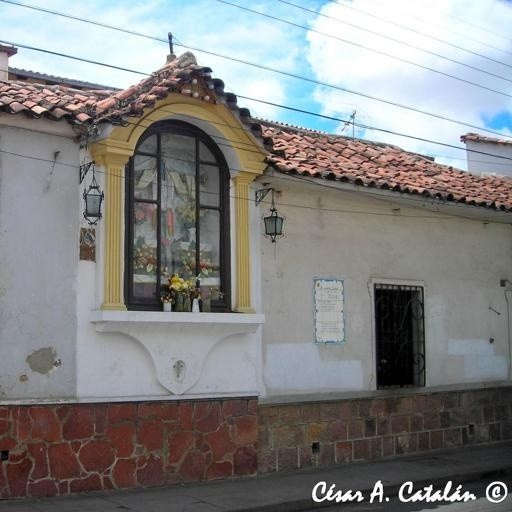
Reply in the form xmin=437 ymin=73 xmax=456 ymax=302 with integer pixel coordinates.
xmin=134 ymin=202 xmax=223 ymax=312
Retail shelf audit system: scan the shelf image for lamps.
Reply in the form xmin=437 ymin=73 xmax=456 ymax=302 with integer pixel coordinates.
xmin=81 ymin=161 xmax=104 ymax=225
xmin=264 ymin=190 xmax=283 ymax=243
